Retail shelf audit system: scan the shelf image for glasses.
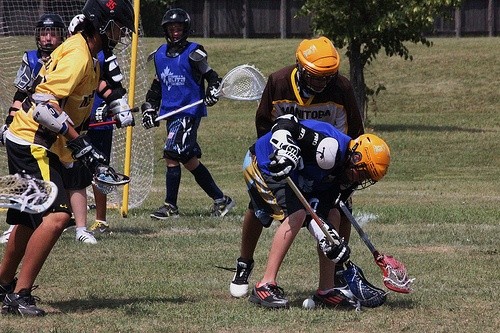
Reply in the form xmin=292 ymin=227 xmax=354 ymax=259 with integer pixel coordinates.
xmin=170 ymin=28 xmax=183 ymax=32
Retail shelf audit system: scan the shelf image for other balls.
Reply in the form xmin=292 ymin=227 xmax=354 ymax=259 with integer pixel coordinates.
xmin=301 ymin=298 xmax=315 ymax=311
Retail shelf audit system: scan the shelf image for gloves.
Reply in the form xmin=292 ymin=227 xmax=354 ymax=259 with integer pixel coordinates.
xmin=105 ymin=91 xmax=135 ymax=129
xmin=0 ymin=115 xmax=14 ymax=147
xmin=330 ymin=179 xmax=358 ymax=210
xmin=307 ymin=215 xmax=351 ymax=264
xmin=65 ymin=133 xmax=109 ymax=175
xmin=203 ymin=78 xmax=222 ymax=107
xmin=94 ymin=100 xmax=110 ymax=123
xmin=268 ymin=129 xmax=302 ymax=181
xmin=141 ymin=102 xmax=159 ymax=129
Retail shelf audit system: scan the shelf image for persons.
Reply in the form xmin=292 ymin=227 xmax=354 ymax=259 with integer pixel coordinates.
xmin=230 ymin=37 xmax=365 ymax=297
xmin=242 ymin=114 xmax=390 ymax=308
xmin=141 ymin=8 xmax=236 ymax=219
xmin=0 ymin=0 xmax=137 ymax=315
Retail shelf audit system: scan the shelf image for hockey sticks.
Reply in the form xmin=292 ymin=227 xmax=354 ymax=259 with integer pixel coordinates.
xmin=154 ymin=62 xmax=268 ymax=122
xmin=0 ymin=107 xmax=139 ymax=147
xmin=284 ymin=176 xmax=415 ymax=307
xmin=0 ymin=172 xmax=58 ymax=214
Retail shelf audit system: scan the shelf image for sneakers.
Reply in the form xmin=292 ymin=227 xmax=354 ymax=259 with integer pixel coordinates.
xmin=210 ymin=195 xmax=235 ymax=218
xmin=88 ymin=219 xmax=113 ymax=239
xmin=75 ymin=227 xmax=98 ymax=244
xmin=229 ymin=257 xmax=254 ymax=298
xmin=313 ymin=288 xmax=360 ymax=310
xmin=0 ymin=278 xmax=18 ymax=302
xmin=149 ymin=201 xmax=180 ymax=219
xmin=1 ymin=288 xmax=45 ymax=316
xmin=247 ymin=280 xmax=290 ymax=308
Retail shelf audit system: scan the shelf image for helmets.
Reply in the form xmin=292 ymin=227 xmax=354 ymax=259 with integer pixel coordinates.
xmin=161 ymin=9 xmax=191 ymax=30
xmin=343 ymin=134 xmax=391 ymax=190
xmin=35 ymin=14 xmax=69 ymax=54
xmin=68 ymin=0 xmax=142 ymax=56
xmin=295 ymin=35 xmax=340 ymax=93
